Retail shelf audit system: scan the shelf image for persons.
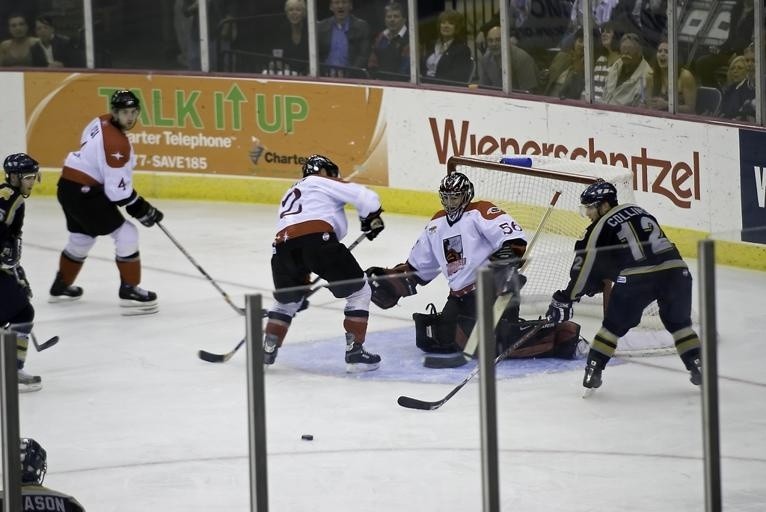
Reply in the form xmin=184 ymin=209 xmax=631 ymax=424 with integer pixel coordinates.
xmin=367 ymin=173 xmax=580 ymax=361
xmin=599 ymin=32 xmax=654 ymax=109
xmin=743 ymin=47 xmax=757 ymax=89
xmin=0 ymin=438 xmax=86 ymax=512
xmin=0 ymin=153 xmax=42 ymax=391
xmin=50 ymin=92 xmax=163 ymax=305
xmin=0 ymin=13 xmax=39 ymax=67
xmin=546 ymin=180 xmax=704 ymax=389
xmin=593 ymin=22 xmax=626 ymax=104
xmin=477 ymin=27 xmax=539 ymax=94
xmin=263 ymin=155 xmax=384 ymax=364
xmin=420 ymin=10 xmax=473 ymax=88
xmin=369 ymin=1 xmax=409 ymax=76
xmin=541 ymin=28 xmax=586 ymax=100
xmin=30 ymin=16 xmax=87 ymax=69
xmin=259 ymin=0 xmax=309 ymax=73
xmin=717 ymin=55 xmax=757 ymax=122
xmin=317 ymin=1 xmax=371 ymax=79
xmin=651 ymin=40 xmax=697 ymax=113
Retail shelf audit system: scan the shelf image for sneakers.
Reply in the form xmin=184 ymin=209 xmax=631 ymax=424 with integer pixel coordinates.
xmin=690 ymin=356 xmax=703 ymax=385
xmin=263 ymin=334 xmax=279 ymax=364
xmin=345 ymin=332 xmax=381 ymax=364
xmin=15 ymin=367 xmax=42 ymax=385
xmin=582 ymin=359 xmax=603 ymax=389
xmin=49 ymin=272 xmax=83 ymax=298
xmin=119 ymin=275 xmax=157 ymax=303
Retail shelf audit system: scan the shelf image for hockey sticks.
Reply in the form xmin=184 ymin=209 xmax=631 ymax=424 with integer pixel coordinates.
xmin=198 ymin=218 xmax=383 ymax=364
xmin=155 ymin=222 xmax=268 ymax=320
xmin=31 ymin=331 xmax=60 ymax=352
xmin=423 ymin=188 xmax=563 ymax=369
xmin=397 ymin=313 xmax=555 ymax=411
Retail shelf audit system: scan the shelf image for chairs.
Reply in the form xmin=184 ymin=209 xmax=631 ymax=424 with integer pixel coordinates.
xmin=468 ymin=57 xmax=476 ymax=83
xmin=695 ymin=87 xmax=722 ymax=117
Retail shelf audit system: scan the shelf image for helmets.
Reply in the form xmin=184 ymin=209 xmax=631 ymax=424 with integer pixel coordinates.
xmin=111 ymin=89 xmax=139 ymax=109
xmin=3 ymin=153 xmax=39 ymax=199
xmin=302 ymin=154 xmax=341 ymax=180
xmin=438 ymin=172 xmax=474 ymax=222
xmin=578 ymin=182 xmax=618 ymax=219
xmin=18 ymin=438 xmax=47 ymax=486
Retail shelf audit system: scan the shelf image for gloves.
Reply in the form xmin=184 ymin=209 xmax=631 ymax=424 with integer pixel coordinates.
xmin=366 ymin=263 xmax=417 ymax=310
xmin=16 ymin=266 xmax=33 ymax=298
xmin=297 ymin=289 xmax=313 ymax=313
xmin=493 ymin=264 xmax=527 ymax=296
xmin=126 ymin=200 xmax=163 ymax=227
xmin=585 ymin=280 xmax=606 ymax=297
xmin=545 ymin=289 xmax=573 ymax=329
xmin=359 ymin=208 xmax=385 ymax=242
